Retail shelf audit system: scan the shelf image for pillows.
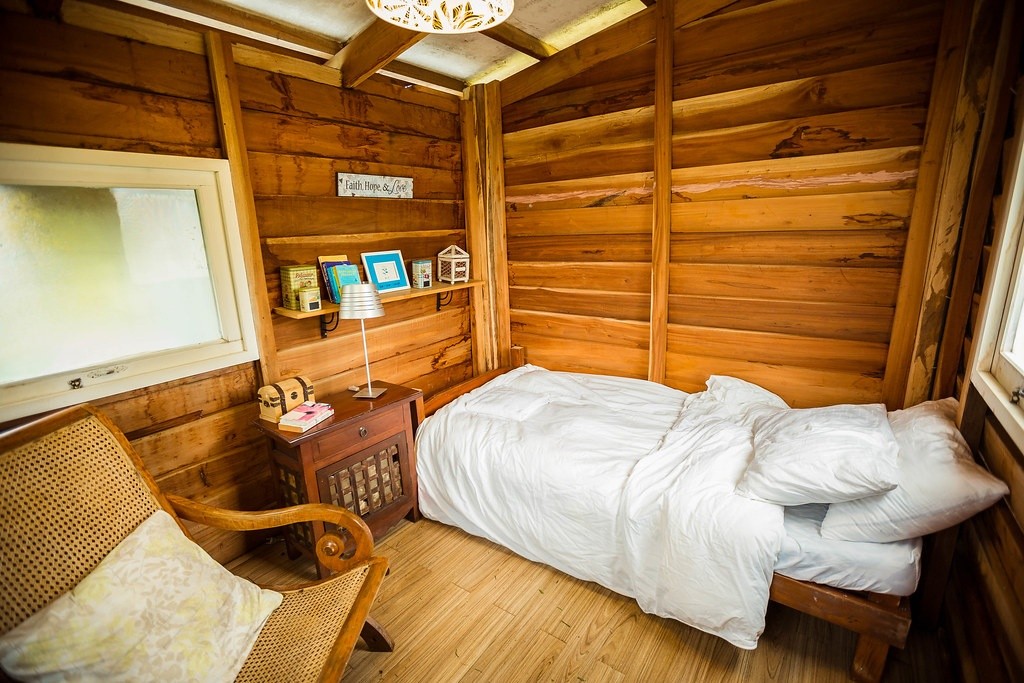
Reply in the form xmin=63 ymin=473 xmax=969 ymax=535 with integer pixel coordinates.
xmin=817 ymin=394 xmax=1011 ymax=543
xmin=734 ymin=401 xmax=900 ymax=505
xmin=0 ymin=509 xmax=284 ymax=683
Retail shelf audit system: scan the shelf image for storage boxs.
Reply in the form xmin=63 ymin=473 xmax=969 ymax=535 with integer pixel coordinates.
xmin=256 ymin=376 xmax=316 ymax=424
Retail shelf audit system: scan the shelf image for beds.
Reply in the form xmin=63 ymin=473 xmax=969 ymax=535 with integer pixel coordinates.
xmin=408 ymin=343 xmax=926 ymax=683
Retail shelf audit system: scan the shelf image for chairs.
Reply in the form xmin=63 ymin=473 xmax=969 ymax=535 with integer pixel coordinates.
xmin=0 ymin=403 xmax=395 ymax=683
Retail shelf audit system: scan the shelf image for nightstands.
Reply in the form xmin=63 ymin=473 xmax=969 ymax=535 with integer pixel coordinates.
xmin=251 ymin=379 xmax=424 ymax=581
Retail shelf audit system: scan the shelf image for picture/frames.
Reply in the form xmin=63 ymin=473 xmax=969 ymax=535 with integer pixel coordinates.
xmin=359 ymin=249 xmax=412 ymax=294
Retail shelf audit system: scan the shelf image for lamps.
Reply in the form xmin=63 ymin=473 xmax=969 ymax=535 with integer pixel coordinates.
xmin=336 ymin=281 xmax=387 ymax=400
xmin=365 ymin=0 xmax=515 ymax=35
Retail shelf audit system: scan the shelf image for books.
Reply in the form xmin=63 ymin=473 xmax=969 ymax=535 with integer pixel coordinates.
xmin=317 ymin=255 xmax=362 ymax=304
xmin=279 ymin=401 xmax=332 ymax=426
xmin=277 ymin=407 xmax=335 ymax=433
